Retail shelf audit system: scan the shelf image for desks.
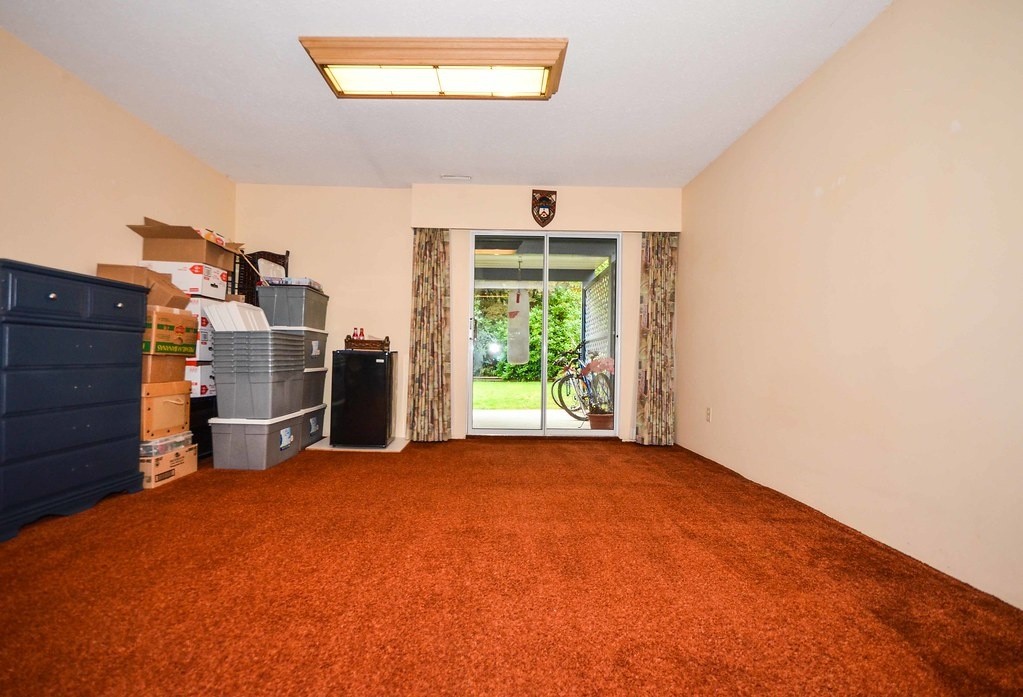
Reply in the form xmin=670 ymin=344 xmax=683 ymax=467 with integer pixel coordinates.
xmin=330 ymin=350 xmax=401 ymax=448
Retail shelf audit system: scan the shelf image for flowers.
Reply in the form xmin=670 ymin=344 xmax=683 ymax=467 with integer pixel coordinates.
xmin=578 ymin=357 xmax=616 ymax=412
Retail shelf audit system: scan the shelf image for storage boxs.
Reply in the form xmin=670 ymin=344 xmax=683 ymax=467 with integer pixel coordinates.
xmin=95 ymin=217 xmax=330 ymax=491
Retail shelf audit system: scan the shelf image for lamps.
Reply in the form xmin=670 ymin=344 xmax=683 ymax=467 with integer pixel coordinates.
xmin=295 ymin=37 xmax=570 ymax=102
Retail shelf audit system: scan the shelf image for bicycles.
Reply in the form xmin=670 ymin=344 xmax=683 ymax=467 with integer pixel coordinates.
xmin=550 ymin=340 xmax=613 ymax=423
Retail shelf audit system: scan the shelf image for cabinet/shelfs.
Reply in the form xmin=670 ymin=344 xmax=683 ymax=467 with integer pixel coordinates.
xmin=1 ymin=259 xmax=149 ymax=538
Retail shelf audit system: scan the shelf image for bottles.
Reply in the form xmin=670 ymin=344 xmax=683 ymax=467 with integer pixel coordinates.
xmin=358 ymin=328 xmax=365 ymax=340
xmin=353 ymin=328 xmax=358 ymax=340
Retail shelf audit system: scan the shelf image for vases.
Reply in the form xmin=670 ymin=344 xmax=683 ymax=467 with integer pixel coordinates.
xmin=585 ymin=412 xmax=614 ymax=431
